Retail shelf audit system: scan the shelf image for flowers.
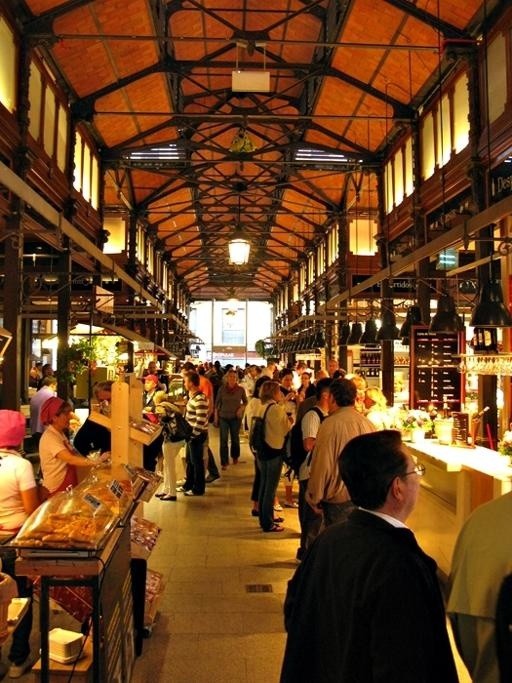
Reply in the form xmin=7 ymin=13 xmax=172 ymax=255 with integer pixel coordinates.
xmin=402 ymin=409 xmax=433 ymax=433
xmin=497 ymin=430 xmax=512 ymax=467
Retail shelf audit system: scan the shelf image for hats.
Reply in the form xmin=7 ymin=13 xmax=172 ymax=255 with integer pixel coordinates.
xmin=0 ymin=409 xmax=26 ymax=447
xmin=143 ymin=374 xmax=158 ymax=383
xmin=40 ymin=396 xmax=65 ymax=424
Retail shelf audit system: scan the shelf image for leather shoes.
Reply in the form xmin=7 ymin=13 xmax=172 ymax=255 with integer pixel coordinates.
xmin=155 ymin=492 xmax=167 ymax=496
xmin=160 ymin=495 xmax=176 ymax=500
xmin=273 ymin=504 xmax=283 ymax=510
xmin=284 ymin=501 xmax=299 ymax=507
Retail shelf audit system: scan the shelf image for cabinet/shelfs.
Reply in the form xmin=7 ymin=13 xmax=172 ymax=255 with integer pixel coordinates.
xmin=89 ymin=373 xmax=168 ymax=638
xmin=353 ymin=348 xmax=380 ymax=388
xmin=0 ymin=482 xmax=149 ymax=683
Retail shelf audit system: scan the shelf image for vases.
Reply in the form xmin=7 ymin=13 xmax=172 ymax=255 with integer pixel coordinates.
xmin=411 ymin=431 xmax=425 ymax=443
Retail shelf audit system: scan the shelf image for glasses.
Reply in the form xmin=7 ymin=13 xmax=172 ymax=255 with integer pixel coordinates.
xmin=401 ymin=462 xmax=426 ymax=475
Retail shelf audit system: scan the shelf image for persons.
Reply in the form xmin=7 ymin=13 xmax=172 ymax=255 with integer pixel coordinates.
xmin=29 ymin=359 xmax=56 ymax=433
xmin=36 ymin=396 xmax=111 ymax=501
xmin=143 ymin=354 xmax=374 ymax=531
xmin=445 ymin=492 xmax=511 ymax=682
xmin=281 ymin=429 xmax=459 ymax=681
xmin=0 ymin=409 xmax=49 ymax=679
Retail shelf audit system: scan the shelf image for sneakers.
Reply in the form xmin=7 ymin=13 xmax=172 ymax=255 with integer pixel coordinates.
xmin=175 ymin=476 xmax=187 ymax=485
xmin=252 ymin=509 xmax=259 ymax=515
xmin=205 ymin=473 xmax=219 ymax=482
xmin=274 ymin=517 xmax=284 ymax=521
xmin=175 ymin=485 xmax=185 ymax=491
xmin=184 ymin=489 xmax=194 ymax=495
xmin=270 ymin=523 xmax=284 ymax=531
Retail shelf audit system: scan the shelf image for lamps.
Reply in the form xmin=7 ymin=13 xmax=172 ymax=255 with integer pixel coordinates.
xmin=222 ymin=286 xmax=245 ymax=315
xmin=40 ymin=258 xmax=59 ymax=283
xmin=228 ymin=192 xmax=251 ymax=265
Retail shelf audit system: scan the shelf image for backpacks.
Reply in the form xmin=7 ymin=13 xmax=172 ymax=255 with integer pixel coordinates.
xmin=283 ymin=407 xmax=325 ymax=470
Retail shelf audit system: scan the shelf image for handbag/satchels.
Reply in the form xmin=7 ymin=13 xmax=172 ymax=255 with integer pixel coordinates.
xmin=249 ymin=402 xmax=276 ymax=461
xmin=160 ymin=411 xmax=193 ymax=441
